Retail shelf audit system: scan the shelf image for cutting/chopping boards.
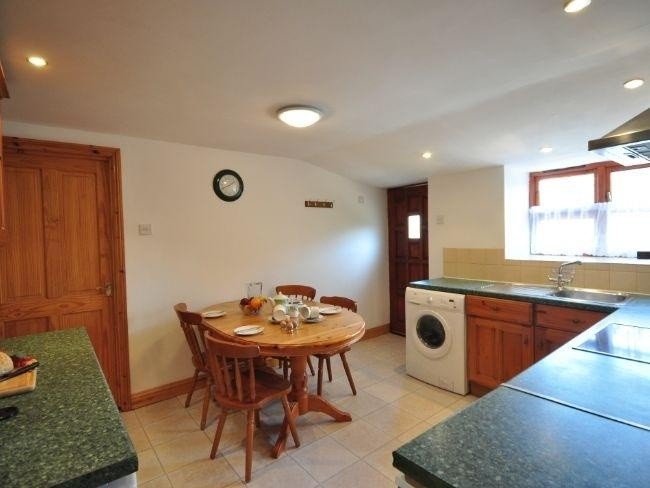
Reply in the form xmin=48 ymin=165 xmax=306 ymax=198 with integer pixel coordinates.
xmin=0 ymin=367 xmax=38 ymax=398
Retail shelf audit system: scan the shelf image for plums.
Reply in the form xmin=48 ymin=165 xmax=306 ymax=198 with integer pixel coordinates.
xmin=241 ymin=299 xmax=249 ymax=305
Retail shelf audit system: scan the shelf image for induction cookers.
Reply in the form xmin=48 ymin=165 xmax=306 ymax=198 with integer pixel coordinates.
xmin=573 ymin=323 xmax=649 ymax=363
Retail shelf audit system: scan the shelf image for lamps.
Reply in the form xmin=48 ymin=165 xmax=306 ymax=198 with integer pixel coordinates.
xmin=276 ymin=107 xmax=325 ymax=128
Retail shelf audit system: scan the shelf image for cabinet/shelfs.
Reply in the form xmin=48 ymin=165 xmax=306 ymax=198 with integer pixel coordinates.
xmin=465 ymin=294 xmax=534 ymax=398
xmin=534 ymin=303 xmax=611 ymax=362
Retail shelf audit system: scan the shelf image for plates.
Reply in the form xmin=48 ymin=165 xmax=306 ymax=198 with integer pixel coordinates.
xmin=318 ymin=305 xmax=342 ymax=314
xmin=302 ymin=315 xmax=324 ymax=323
xmin=201 ymin=310 xmax=226 ymax=318
xmin=234 ymin=324 xmax=264 ymax=336
xmin=269 ymin=316 xmax=289 ymax=323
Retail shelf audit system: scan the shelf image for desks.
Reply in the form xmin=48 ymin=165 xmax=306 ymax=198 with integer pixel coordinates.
xmin=1 ymin=326 xmax=139 ymax=488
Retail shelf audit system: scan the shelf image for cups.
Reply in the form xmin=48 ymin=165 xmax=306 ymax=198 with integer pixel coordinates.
xmin=301 ymin=306 xmax=319 ymax=319
xmin=273 ymin=305 xmax=286 ymax=322
xmin=286 ymin=297 xmax=305 ymax=318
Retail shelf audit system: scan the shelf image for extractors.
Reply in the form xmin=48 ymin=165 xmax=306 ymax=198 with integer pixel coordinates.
xmin=588 ymin=106 xmax=650 ymax=168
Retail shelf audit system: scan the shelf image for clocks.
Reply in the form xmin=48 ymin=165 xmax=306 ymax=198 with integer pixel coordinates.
xmin=214 ymin=169 xmax=244 ymax=201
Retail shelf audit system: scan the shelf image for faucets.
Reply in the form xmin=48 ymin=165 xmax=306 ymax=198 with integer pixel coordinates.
xmin=548 ymin=260 xmax=582 ymax=287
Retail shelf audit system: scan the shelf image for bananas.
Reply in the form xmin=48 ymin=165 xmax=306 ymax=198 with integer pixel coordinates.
xmin=256 ymin=296 xmax=267 ymax=303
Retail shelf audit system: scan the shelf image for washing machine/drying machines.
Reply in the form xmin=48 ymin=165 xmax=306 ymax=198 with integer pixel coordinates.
xmin=404 ymin=287 xmax=471 ymax=396
xmin=543 ymin=288 xmax=635 ymax=305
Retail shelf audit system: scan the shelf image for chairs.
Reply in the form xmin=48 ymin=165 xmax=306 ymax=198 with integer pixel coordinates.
xmin=204 ymin=330 xmax=301 ymax=485
xmin=275 ymin=284 xmax=316 ymax=382
xmin=174 ymin=302 xmax=250 ymax=431
xmin=307 ymin=296 xmax=358 ymax=398
